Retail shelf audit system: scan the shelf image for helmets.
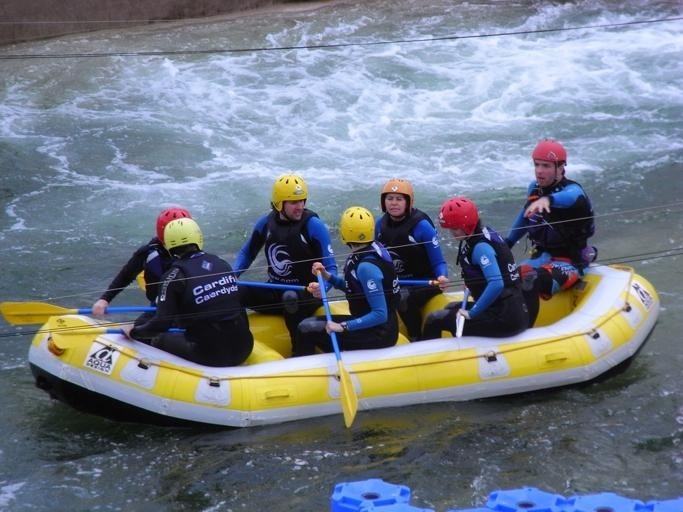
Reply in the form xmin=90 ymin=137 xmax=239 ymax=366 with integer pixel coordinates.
xmin=340 ymin=206 xmax=376 ymax=245
xmin=532 ymin=140 xmax=567 ymax=163
xmin=271 ymin=176 xmax=308 ymax=211
xmin=438 ymin=198 xmax=479 ymax=236
xmin=379 ymin=179 xmax=415 ymax=214
xmin=164 ymin=217 xmax=205 ymax=254
xmin=156 ymin=208 xmax=192 ymax=241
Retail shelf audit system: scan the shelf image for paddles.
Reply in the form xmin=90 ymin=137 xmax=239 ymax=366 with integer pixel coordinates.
xmin=137 ymin=271 xmax=315 ymax=294
xmin=0 ymin=301 xmax=158 ymax=326
xmin=316 ymin=271 xmax=360 ymax=428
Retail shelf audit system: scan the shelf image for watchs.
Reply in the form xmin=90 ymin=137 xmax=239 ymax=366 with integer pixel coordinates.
xmin=339 ymin=319 xmax=348 ymax=330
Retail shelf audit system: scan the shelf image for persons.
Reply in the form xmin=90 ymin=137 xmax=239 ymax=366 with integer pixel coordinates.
xmin=502 ymin=138 xmax=597 ymax=328
xmin=231 ymin=173 xmax=338 ymax=356
xmin=120 ymin=217 xmax=255 ymax=366
xmin=290 ymin=205 xmax=402 ymax=357
xmin=373 ymin=176 xmax=450 ymax=343
xmin=420 ymin=196 xmax=530 ymax=341
xmin=91 ymin=206 xmax=191 ymax=325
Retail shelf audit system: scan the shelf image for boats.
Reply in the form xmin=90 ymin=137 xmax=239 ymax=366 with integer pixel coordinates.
xmin=27 ymin=259 xmax=660 ymax=431
